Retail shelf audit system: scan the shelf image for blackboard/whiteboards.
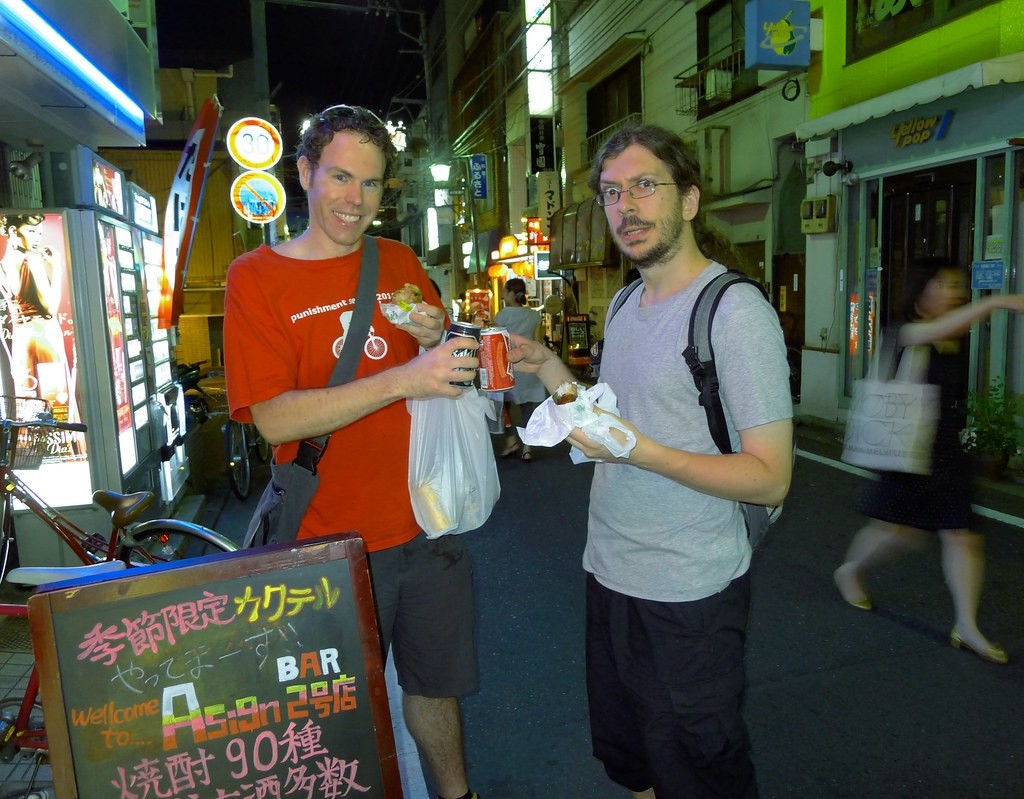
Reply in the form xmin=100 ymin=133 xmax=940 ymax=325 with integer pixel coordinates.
xmin=565 ymin=313 xmax=592 ymax=366
xmin=26 ymin=530 xmax=402 ymax=799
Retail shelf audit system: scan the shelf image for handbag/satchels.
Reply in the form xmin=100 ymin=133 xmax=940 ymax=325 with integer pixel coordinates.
xmin=242 ymin=458 xmax=320 ymax=550
xmin=403 ymin=326 xmax=501 ymax=541
xmin=840 ymin=321 xmax=941 ymax=477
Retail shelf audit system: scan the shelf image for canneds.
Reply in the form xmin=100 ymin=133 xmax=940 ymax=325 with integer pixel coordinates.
xmin=477 ymin=326 xmax=515 ymax=391
xmin=444 ymin=322 xmax=481 ymax=388
xmin=413 ymin=476 xmax=459 ymax=538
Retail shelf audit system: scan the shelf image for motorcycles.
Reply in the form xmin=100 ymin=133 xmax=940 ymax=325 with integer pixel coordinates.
xmin=177 ymin=359 xmax=210 ymax=425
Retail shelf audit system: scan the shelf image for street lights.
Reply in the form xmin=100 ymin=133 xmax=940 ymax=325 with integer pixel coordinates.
xmin=429 ymin=156 xmax=483 ymax=291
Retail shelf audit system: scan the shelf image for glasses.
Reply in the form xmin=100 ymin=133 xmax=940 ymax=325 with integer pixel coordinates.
xmin=592 ymin=181 xmax=679 ymax=207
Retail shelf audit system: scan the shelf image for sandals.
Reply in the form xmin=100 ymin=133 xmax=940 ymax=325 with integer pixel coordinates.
xmin=494 ymin=443 xmax=520 ymax=457
xmin=521 ymin=452 xmax=534 ymax=461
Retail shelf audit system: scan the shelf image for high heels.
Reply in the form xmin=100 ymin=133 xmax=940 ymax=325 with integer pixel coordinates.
xmin=833 ymin=570 xmax=873 ymax=610
xmin=950 ymin=629 xmax=1008 ymax=664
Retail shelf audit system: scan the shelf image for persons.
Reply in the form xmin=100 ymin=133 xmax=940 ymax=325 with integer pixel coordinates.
xmin=223 ymin=104 xmax=480 ymax=798
xmin=836 ymin=257 xmax=1024 ymax=664
xmin=503 ymin=124 xmax=795 ymax=799
xmin=0 ymin=215 xmax=71 ymax=404
xmin=494 ymin=278 xmax=543 ymax=462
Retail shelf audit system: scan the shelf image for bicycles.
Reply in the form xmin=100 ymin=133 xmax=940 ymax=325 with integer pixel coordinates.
xmin=0 ymin=395 xmax=243 ymax=593
xmin=196 ymin=370 xmax=270 ymax=504
xmin=0 ymin=559 xmax=126 ymax=799
xmin=463 ymin=309 xmax=495 ymax=328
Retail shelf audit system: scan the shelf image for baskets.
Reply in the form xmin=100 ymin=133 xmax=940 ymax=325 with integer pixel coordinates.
xmin=0 ymin=395 xmax=54 ymax=470
